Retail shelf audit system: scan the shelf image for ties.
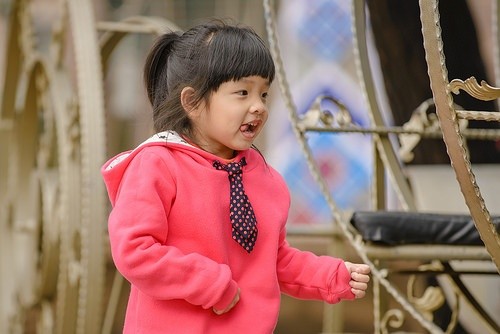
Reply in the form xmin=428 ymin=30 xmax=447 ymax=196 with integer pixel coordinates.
xmin=213 ymin=157 xmax=259 ymax=255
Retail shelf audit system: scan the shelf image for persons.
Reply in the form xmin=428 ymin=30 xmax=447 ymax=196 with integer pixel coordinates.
xmin=101 ymin=17 xmax=371 ymax=333
